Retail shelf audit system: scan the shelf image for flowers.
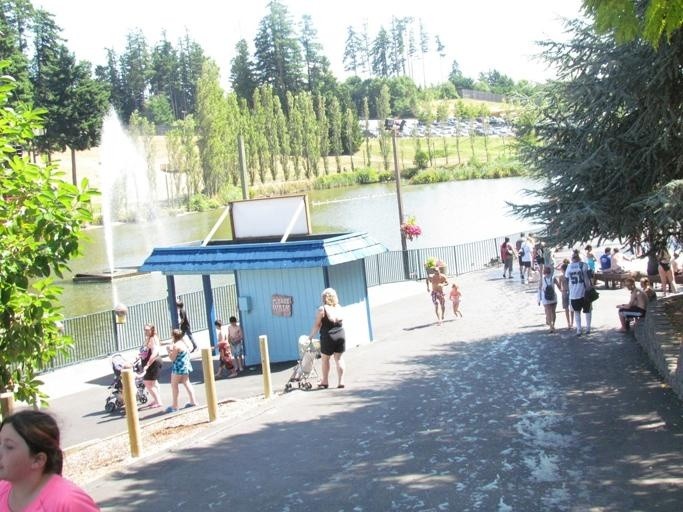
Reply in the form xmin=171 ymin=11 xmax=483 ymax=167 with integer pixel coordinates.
xmin=401 ymin=214 xmax=421 ymax=238
xmin=425 ymin=256 xmax=446 ymax=268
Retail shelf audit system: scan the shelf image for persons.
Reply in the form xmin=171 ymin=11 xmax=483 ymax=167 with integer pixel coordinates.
xmin=501 ymin=217 xmax=683 ymax=336
xmin=426 ymin=267 xmax=448 ymax=325
xmin=0 ymin=410 xmax=102 ymax=512
xmin=140 ymin=325 xmax=161 ymax=410
xmin=120 ymin=365 xmax=146 ymax=410
xmin=214 ymin=316 xmax=244 ymax=380
xmin=449 ymin=283 xmax=463 ymax=317
xmin=165 ymin=328 xmax=196 ymax=413
xmin=308 ymin=287 xmax=345 ymax=388
xmin=176 ymin=302 xmax=197 ymax=353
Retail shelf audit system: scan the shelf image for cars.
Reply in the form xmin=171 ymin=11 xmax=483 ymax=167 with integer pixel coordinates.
xmin=357 ymin=115 xmax=523 ymax=137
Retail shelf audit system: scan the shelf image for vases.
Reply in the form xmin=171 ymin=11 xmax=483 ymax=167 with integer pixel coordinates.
xmin=426 ymin=268 xmax=445 ymax=278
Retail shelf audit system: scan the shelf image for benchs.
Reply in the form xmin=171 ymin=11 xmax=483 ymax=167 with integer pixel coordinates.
xmin=648 ymin=271 xmax=683 ymax=285
xmin=591 ymin=273 xmax=635 ymax=289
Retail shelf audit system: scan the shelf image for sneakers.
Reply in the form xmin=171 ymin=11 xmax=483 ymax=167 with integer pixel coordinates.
xmin=146 ymin=402 xmax=196 ymax=413
xmin=317 ymin=384 xmax=345 ymax=388
xmin=215 ymin=368 xmax=244 ymax=379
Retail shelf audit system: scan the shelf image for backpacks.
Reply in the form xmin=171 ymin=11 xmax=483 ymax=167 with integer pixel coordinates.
xmin=543 ymin=277 xmax=554 ymax=300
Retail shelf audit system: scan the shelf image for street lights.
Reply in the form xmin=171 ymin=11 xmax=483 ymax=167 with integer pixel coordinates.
xmin=383 ymin=118 xmax=412 ymax=279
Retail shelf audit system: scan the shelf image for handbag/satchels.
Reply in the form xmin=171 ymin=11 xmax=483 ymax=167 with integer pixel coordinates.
xmin=583 ymin=289 xmax=599 ymax=303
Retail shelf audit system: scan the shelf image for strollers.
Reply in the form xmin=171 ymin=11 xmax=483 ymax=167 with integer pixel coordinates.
xmin=103 ymin=353 xmax=148 ymax=415
xmin=284 ymin=335 xmax=324 ymax=392
